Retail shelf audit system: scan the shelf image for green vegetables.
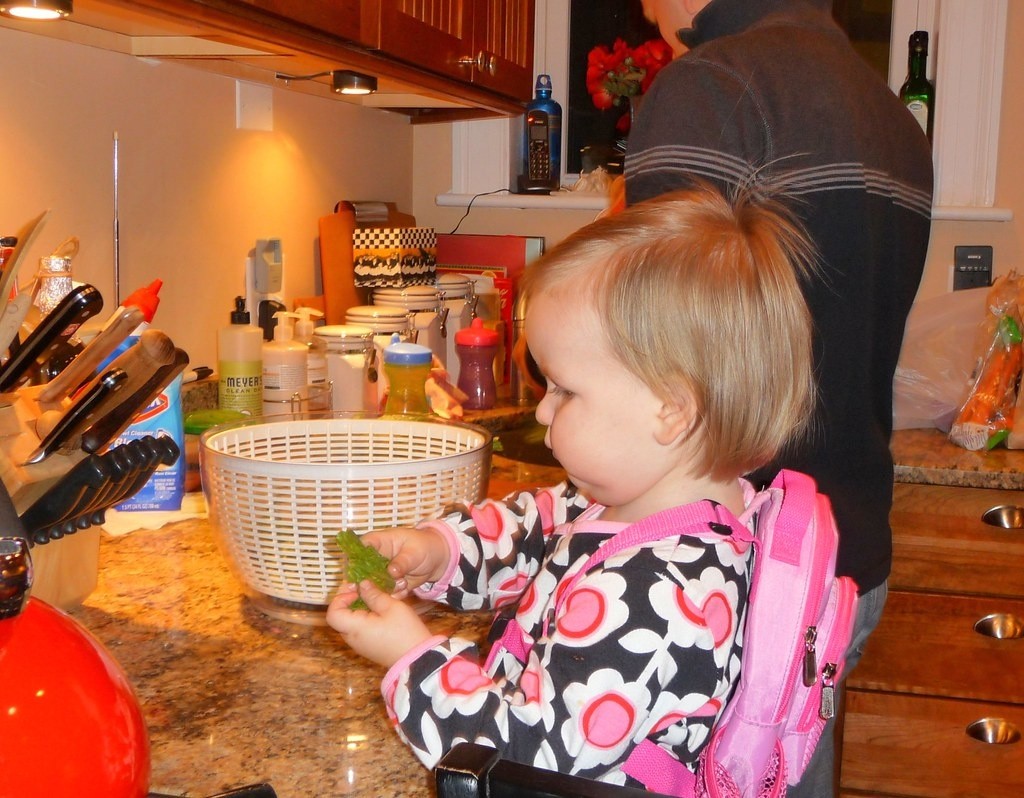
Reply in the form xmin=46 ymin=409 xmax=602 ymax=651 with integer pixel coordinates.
xmin=333 ymin=527 xmax=398 ymax=611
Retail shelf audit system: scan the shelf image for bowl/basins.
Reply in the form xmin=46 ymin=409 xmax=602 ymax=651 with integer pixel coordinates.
xmin=182 ymin=407 xmax=252 ymax=492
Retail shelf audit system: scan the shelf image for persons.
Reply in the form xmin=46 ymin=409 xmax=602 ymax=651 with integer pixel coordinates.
xmin=623 ymin=0 xmax=935 ymax=798
xmin=324 ymin=185 xmax=815 ymax=798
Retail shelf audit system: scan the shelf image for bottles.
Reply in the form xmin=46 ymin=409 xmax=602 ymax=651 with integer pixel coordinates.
xmin=217 ymin=296 xmax=264 ymax=423
xmin=523 ymin=73 xmax=562 ymax=190
xmin=901 ymin=30 xmax=935 ymax=141
xmin=383 ymin=332 xmax=434 ymax=416
xmin=453 ymin=317 xmax=500 ymax=409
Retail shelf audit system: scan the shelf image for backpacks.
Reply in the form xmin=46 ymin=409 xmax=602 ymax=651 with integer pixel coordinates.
xmin=554 ymin=469 xmax=858 ymax=798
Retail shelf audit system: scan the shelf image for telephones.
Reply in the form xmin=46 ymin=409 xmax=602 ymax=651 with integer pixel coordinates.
xmin=517 ymin=107 xmax=559 ymax=194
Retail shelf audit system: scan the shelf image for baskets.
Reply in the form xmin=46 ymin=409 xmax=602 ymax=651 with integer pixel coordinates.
xmin=202 ymin=418 xmax=489 ymax=605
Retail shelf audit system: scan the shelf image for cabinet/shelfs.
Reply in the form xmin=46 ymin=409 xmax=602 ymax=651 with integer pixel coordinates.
xmin=829 ymin=482 xmax=1024 ymax=798
xmin=0 ymin=0 xmax=536 ymax=127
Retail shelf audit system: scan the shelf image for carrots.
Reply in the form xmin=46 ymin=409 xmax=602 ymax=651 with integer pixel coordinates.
xmin=956 ymin=337 xmax=1024 ymax=441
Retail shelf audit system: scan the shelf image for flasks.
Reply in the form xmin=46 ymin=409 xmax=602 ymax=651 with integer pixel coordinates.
xmin=511 ymin=289 xmax=538 ymax=407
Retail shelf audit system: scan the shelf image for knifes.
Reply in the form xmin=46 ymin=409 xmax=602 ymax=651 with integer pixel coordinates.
xmin=1 ymin=210 xmax=190 ymax=548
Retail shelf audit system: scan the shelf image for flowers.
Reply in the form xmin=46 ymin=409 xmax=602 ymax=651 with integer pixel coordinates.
xmin=587 ymin=37 xmax=675 ymax=132
